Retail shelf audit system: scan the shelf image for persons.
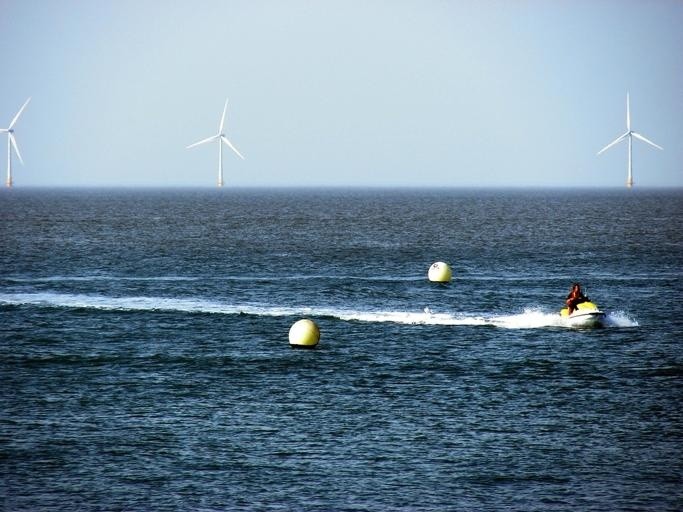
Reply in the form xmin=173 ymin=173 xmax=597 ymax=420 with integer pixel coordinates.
xmin=566 ymin=283 xmax=590 ymax=315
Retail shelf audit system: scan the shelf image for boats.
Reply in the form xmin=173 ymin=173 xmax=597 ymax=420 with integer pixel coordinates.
xmin=558 ymin=301 xmax=604 ymax=329
